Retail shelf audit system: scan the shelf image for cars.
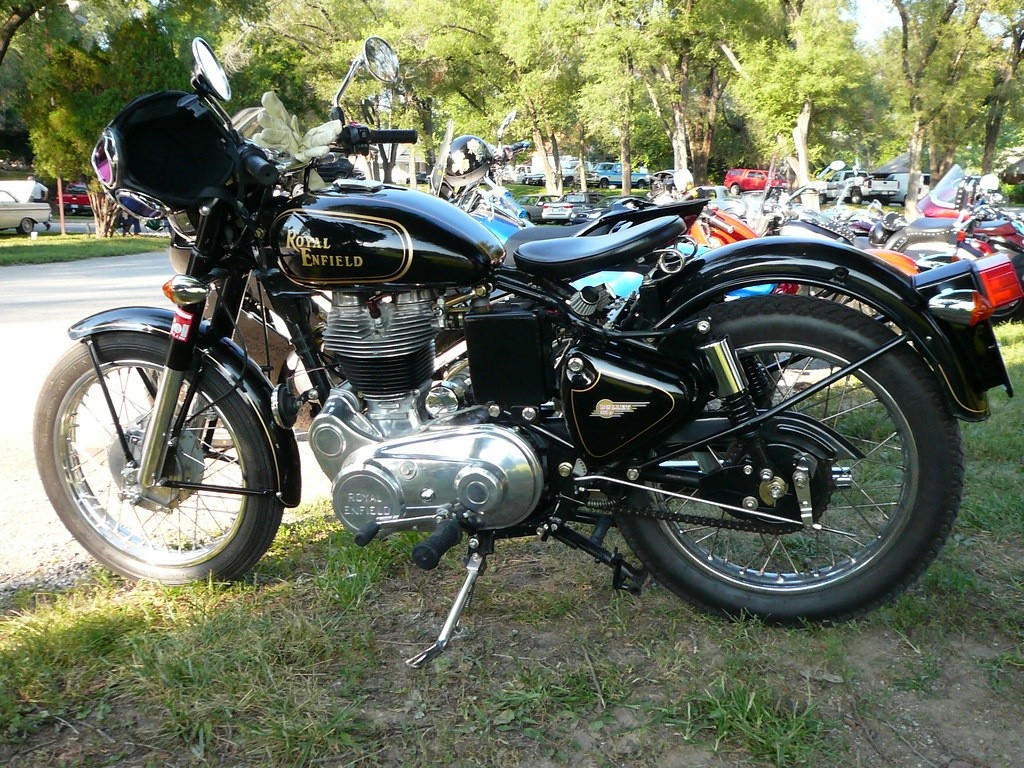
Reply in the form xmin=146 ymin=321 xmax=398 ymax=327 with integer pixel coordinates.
xmin=964 ymin=176 xmax=986 ymax=193
xmin=416 ymin=173 xmax=428 ymax=185
xmin=424 ymin=174 xmax=432 ymax=184
xmin=0 ymin=181 xmax=52 ymax=233
xmin=680 ymin=185 xmax=730 ymax=203
xmin=54 ymin=182 xmax=92 ymax=217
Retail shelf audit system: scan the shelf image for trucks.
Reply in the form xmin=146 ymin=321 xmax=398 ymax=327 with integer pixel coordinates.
xmin=819 ymin=170 xmax=901 ymax=207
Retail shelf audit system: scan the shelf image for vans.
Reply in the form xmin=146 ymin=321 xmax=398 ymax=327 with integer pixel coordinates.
xmin=884 ymin=173 xmax=932 ymax=207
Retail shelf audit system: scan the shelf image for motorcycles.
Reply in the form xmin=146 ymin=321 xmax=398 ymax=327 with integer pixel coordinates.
xmin=431 ymin=108 xmax=1024 ymax=384
xmin=33 ymin=35 xmax=1018 ymax=670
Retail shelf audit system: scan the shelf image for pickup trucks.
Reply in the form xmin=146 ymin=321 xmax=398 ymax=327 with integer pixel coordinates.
xmin=724 ymin=168 xmax=783 ymax=196
xmin=585 ymin=162 xmax=655 ymax=190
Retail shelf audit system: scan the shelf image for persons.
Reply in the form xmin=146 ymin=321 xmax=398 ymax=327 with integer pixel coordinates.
xmin=122 ymin=215 xmax=142 ymax=238
xmin=0 ymin=158 xmax=37 ymax=172
xmin=28 ymin=176 xmax=51 ymax=231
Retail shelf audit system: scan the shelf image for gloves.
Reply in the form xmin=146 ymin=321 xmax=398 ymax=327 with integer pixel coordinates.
xmin=253 ymin=90 xmax=346 ymax=192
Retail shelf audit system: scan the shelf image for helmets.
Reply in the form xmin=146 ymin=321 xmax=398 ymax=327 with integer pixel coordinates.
xmin=650 ymin=169 xmax=693 ymax=200
xmin=90 ymin=90 xmax=240 ymax=220
xmin=868 ymin=221 xmax=892 ymax=246
xmin=444 ymin=136 xmax=493 ymax=185
xmin=980 ymin=173 xmax=1000 ymax=190
